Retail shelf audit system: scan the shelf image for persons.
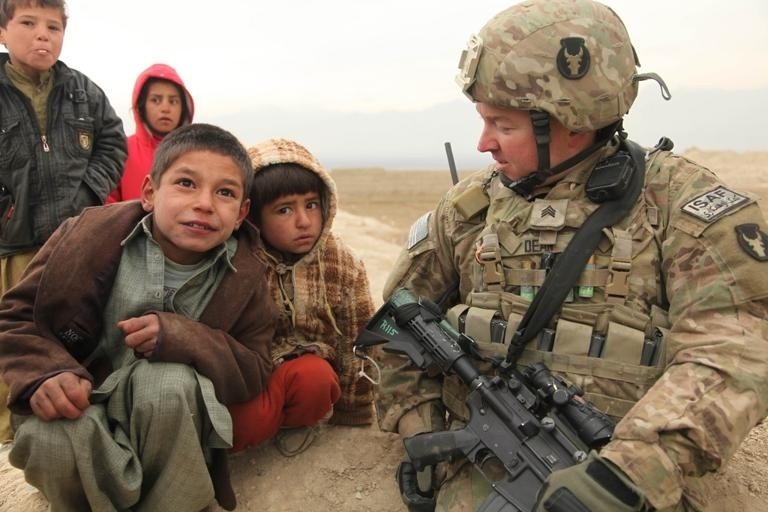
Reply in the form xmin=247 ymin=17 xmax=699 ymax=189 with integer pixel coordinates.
xmin=0 ymin=0 xmax=127 ymax=448
xmin=104 ymin=63 xmax=197 ymax=206
xmin=226 ymin=140 xmax=381 ymax=459
xmin=371 ymin=2 xmax=767 ymax=512
xmin=1 ymin=124 xmax=285 ymax=512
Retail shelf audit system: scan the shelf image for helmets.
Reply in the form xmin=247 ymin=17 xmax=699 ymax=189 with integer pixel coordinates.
xmin=455 ymin=0 xmax=641 ymax=132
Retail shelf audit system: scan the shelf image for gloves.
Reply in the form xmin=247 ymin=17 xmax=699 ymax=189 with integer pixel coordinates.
xmin=532 ymin=449 xmax=656 ymax=512
xmin=396 ymin=445 xmax=436 ymax=511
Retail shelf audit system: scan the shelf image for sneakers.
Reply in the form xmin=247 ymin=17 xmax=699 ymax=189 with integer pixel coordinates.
xmin=276 ymin=424 xmax=317 ymax=457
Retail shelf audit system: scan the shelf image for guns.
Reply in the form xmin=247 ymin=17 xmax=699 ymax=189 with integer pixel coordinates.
xmin=353 ymin=287 xmax=617 ymax=512
